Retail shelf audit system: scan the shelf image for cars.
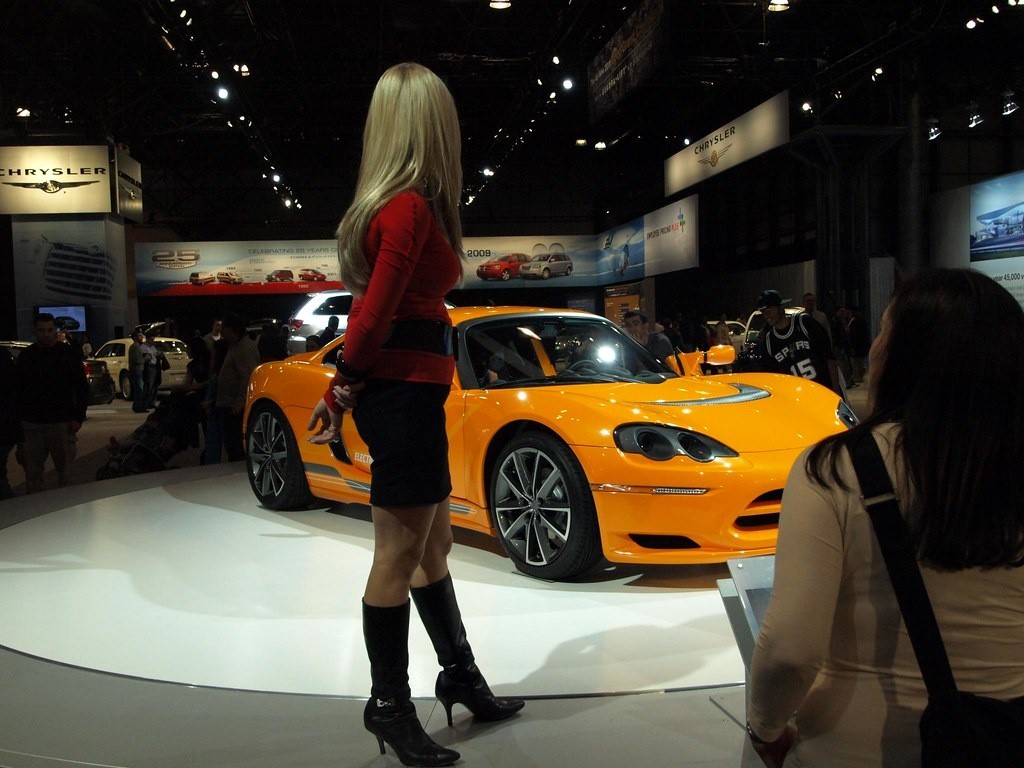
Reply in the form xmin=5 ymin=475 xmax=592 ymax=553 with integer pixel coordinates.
xmin=704 ymin=306 xmax=801 ymax=355
xmin=476 ymin=253 xmax=530 ymax=282
xmin=517 ymin=252 xmax=572 ymax=281
xmin=298 ymin=268 xmax=327 ymax=282
xmin=0 ymin=339 xmax=117 ymax=407
xmin=86 ymin=335 xmax=193 ymax=401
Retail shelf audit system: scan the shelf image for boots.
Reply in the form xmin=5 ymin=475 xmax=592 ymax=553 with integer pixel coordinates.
xmin=358 ymin=597 xmax=463 ymax=767
xmin=408 ymin=573 xmax=525 ymax=727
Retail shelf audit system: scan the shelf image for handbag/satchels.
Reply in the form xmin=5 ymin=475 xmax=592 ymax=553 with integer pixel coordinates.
xmin=918 ymin=689 xmax=1024 ymax=768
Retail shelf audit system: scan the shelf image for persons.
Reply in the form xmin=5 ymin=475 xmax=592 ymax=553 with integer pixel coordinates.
xmin=307 ymin=62 xmax=525 ymax=767
xmin=746 ymin=289 xmax=842 ymax=398
xmin=746 ymin=269 xmax=1024 ymax=768
xmin=129 ymin=330 xmax=163 ymax=413
xmin=663 ymin=311 xmax=732 ymax=374
xmin=830 ymin=305 xmax=871 ymax=389
xmin=107 ymin=406 xmax=177 ymax=457
xmin=625 ymin=311 xmax=681 ymax=375
xmin=803 ymin=293 xmax=831 ymax=347
xmin=306 ymin=316 xmax=339 ymax=352
xmin=184 ymin=320 xmax=290 ymax=465
xmin=0 ymin=313 xmax=93 ymax=499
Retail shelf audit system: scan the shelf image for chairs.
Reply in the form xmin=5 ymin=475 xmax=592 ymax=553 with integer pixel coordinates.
xmin=497 ymin=342 xmax=542 ymax=385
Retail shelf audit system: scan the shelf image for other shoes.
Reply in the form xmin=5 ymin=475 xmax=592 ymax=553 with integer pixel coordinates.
xmin=131 ymin=403 xmax=156 ymax=413
xmin=106 ymin=435 xmax=121 ymax=456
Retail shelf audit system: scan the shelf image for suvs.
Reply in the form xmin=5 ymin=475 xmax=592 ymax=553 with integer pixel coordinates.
xmin=285 ymin=289 xmax=459 ymax=357
xmin=189 ymin=272 xmax=216 ymax=287
xmin=217 ymin=271 xmax=243 ymax=285
xmin=266 ymin=270 xmax=294 ymax=282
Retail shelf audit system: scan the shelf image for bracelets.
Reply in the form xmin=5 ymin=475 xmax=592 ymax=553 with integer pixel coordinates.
xmin=336 ymin=356 xmax=368 ymax=378
xmin=746 ymin=721 xmax=766 ymax=745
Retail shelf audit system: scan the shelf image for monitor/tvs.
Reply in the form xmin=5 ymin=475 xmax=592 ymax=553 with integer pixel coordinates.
xmin=37 ymin=305 xmax=86 ymax=333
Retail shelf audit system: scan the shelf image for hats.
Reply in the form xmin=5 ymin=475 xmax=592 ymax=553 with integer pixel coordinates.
xmin=755 ymin=288 xmax=793 ymax=311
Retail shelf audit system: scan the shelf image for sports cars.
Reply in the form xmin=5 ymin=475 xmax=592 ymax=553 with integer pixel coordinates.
xmin=240 ymin=304 xmax=861 ymax=585
